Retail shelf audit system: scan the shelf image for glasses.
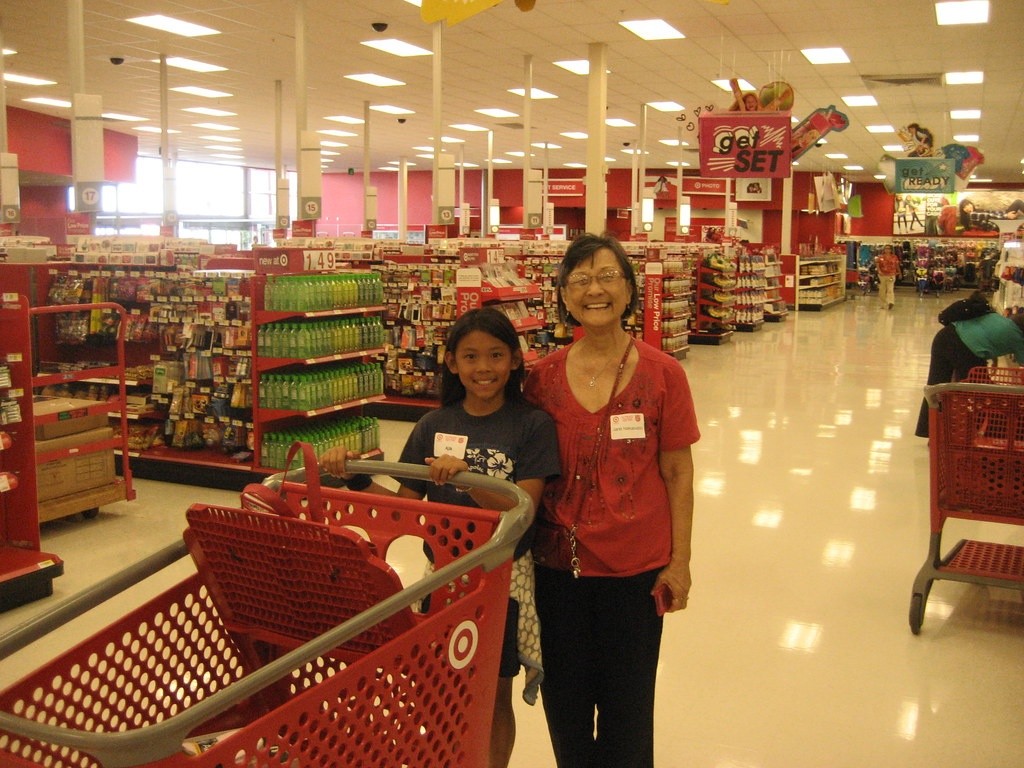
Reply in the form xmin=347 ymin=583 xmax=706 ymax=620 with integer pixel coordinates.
xmin=566 ymin=268 xmax=624 ymax=288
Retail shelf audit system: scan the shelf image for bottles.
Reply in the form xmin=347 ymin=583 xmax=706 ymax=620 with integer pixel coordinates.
xmin=260 ymin=412 xmax=380 ymax=470
xmin=256 ymin=314 xmax=384 ymax=359
xmin=662 ymin=298 xmax=688 ymax=315
xmin=258 ymin=360 xmax=383 ymax=411
xmin=663 ymin=278 xmax=690 ymax=293
xmin=661 ymin=316 xmax=687 ymax=334
xmin=662 ymin=334 xmax=688 ymax=351
xmin=264 ymin=273 xmax=383 ymax=311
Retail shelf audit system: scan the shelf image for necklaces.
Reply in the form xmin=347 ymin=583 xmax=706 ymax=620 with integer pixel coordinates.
xmin=582 ymin=332 xmax=627 ymax=387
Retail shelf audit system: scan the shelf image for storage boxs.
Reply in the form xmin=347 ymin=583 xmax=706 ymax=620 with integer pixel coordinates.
xmin=34 ymin=428 xmax=116 ymax=504
xmin=33 ymin=398 xmax=109 ymax=440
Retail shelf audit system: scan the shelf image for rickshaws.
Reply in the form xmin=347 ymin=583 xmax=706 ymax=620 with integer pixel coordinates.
xmin=28 ymin=303 xmax=136 ymax=523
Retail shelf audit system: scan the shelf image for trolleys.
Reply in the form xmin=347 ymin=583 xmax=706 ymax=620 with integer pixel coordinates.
xmin=0 ymin=464 xmax=536 ymax=768
xmin=909 ymin=367 xmax=1024 ymax=635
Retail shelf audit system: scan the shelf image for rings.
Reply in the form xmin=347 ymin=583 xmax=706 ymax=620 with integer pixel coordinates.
xmin=672 ymin=597 xmax=683 ymax=601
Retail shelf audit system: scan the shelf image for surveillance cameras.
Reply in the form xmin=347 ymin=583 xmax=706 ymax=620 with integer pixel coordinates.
xmin=398 ymin=119 xmax=406 ymax=123
xmin=624 ymin=142 xmax=630 ymax=147
xmin=372 ymin=23 xmax=387 ymax=33
xmin=111 ymin=57 xmax=124 ymax=66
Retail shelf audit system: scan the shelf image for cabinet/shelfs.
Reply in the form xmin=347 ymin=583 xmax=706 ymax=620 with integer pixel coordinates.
xmin=0 ymin=244 xmax=386 ymax=478
xmin=335 ymin=244 xmax=543 ymax=409
xmin=688 ymin=242 xmax=847 ymax=346
xmin=511 ymin=241 xmax=695 ymax=366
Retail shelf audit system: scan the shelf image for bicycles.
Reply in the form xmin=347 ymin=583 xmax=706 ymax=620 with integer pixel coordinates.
xmin=914 ymin=258 xmax=960 ymax=298
xmin=853 ymin=261 xmax=875 ymax=296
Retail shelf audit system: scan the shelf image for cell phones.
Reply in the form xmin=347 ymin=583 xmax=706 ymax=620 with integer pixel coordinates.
xmin=654 ymin=583 xmax=671 ymax=616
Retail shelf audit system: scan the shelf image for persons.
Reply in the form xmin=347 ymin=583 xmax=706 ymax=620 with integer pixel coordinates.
xmin=915 ymin=307 xmax=1024 ymax=448
xmin=319 ymin=306 xmax=562 ymax=768
xmin=522 ymin=232 xmax=701 ymax=768
xmin=729 ymin=78 xmax=758 ymax=111
xmin=896 ymin=194 xmax=1024 ymax=234
xmin=875 ymin=245 xmax=902 ymax=310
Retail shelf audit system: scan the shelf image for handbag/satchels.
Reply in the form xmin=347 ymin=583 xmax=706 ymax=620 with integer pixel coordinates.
xmin=939 ymin=293 xmax=994 ymax=326
